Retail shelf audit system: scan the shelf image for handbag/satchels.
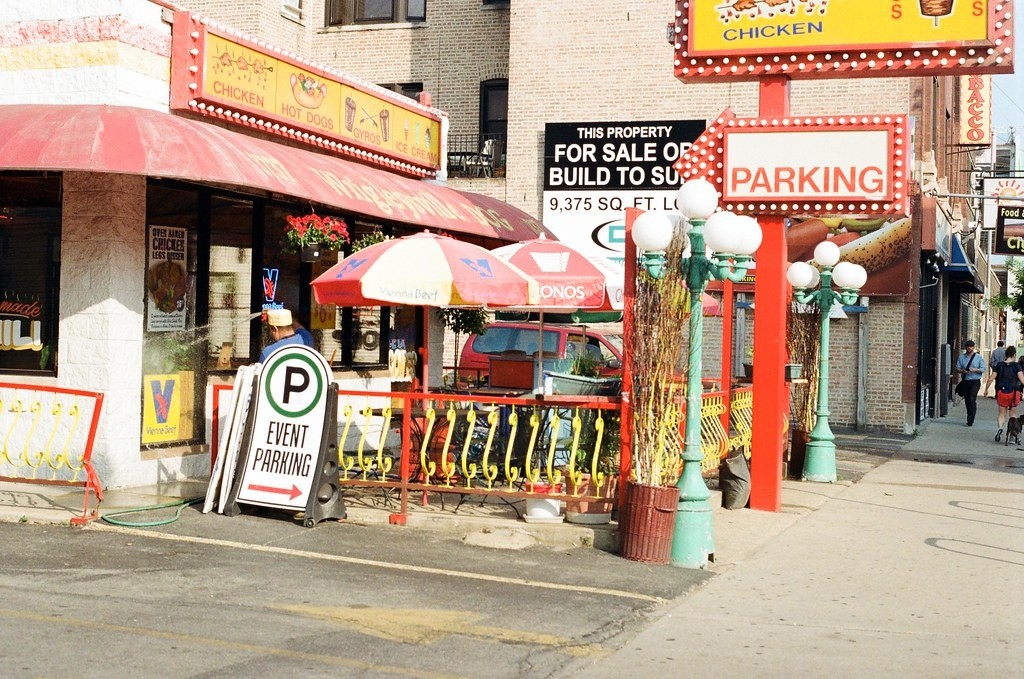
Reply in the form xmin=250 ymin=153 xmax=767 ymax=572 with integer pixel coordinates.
xmin=955 ymin=379 xmax=966 ymax=397
xmin=1001 ymin=383 xmax=1013 ymax=392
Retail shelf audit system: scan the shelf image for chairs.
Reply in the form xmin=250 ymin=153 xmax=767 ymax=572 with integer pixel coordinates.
xmin=455 ymin=393 xmax=547 ymax=520
xmin=337 ymin=448 xmax=396 ymax=510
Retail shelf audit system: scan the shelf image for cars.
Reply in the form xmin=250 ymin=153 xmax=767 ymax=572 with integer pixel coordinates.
xmin=458 ymin=319 xmax=689 ymax=400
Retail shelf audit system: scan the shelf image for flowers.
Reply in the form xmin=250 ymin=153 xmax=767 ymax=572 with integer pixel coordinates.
xmin=279 ymin=214 xmax=350 ymax=252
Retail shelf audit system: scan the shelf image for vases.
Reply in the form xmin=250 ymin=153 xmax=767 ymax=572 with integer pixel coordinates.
xmin=524 ymin=480 xmax=563 ymax=517
xmin=300 ymin=243 xmax=323 ymax=261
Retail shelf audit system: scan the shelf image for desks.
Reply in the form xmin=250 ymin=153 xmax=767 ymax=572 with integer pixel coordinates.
xmin=357 ymin=408 xmax=489 ymax=512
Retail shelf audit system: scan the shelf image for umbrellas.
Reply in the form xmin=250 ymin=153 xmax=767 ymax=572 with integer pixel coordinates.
xmin=309 ymin=229 xmax=540 ymax=507
xmin=441 ymin=231 xmax=624 ymax=482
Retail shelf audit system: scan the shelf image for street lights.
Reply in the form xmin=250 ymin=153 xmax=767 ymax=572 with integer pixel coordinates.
xmin=630 ymin=177 xmax=762 ymax=571
xmin=786 ymin=240 xmax=868 ymax=483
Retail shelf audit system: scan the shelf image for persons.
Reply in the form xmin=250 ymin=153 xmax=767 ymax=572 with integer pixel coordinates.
xmin=956 ymin=340 xmax=986 ymax=426
xmin=1017 ymin=356 xmax=1024 ymax=401
xmin=984 ymin=346 xmax=1024 ymax=444
xmin=989 ymin=341 xmax=1005 ymax=398
xmin=260 ymin=308 xmax=313 ymax=363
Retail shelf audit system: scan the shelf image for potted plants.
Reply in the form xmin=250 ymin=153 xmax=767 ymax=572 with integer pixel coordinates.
xmin=785 ymin=295 xmax=821 ymax=480
xmin=564 ymin=347 xmax=623 ymax=525
xmin=618 ymin=219 xmax=712 ymax=564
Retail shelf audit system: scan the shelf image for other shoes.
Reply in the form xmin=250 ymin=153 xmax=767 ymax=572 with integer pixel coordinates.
xmin=966 ymin=421 xmax=973 ymax=426
xmin=1009 ymin=436 xmax=1015 ymax=443
xmin=994 ymin=428 xmax=1003 ymax=442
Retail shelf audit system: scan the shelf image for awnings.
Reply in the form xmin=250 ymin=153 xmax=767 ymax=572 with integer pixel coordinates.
xmin=948 ymin=234 xmax=985 ymax=294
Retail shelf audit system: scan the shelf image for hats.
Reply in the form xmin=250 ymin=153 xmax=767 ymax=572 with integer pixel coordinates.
xmin=267 ymin=309 xmax=292 ymax=326
xmin=965 ymin=340 xmax=974 ymax=346
